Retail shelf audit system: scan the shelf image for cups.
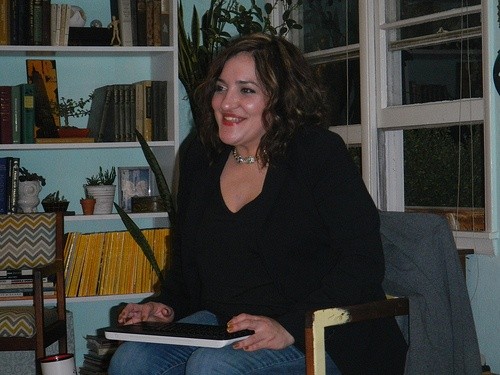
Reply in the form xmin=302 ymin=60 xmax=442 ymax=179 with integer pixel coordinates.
xmin=40 ymin=354 xmax=77 ymax=375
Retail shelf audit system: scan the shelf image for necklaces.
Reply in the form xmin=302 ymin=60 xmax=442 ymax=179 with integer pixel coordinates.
xmin=230 ymin=146 xmax=274 ymax=163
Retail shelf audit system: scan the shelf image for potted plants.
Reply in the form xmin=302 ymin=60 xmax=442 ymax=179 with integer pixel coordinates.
xmin=80 ymin=195 xmax=97 ymax=215
xmin=82 ymin=166 xmax=118 ymax=214
xmin=17 ymin=166 xmax=46 ymax=213
xmin=41 ymin=190 xmax=70 ymax=212
xmin=50 ymin=92 xmax=95 ymax=138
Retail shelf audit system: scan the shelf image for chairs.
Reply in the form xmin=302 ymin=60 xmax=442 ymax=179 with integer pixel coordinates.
xmin=304 ymin=210 xmax=482 ymax=375
xmin=0 ymin=213 xmax=69 ymax=375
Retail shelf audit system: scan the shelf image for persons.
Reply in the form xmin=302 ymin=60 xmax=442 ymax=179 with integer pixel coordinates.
xmin=106 ymin=32 xmax=407 ymax=375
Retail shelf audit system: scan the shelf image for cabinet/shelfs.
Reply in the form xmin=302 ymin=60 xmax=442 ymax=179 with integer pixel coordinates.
xmin=0 ymin=0 xmax=180 ymax=307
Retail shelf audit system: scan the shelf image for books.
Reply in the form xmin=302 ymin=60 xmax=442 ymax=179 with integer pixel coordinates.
xmin=85 ymin=80 xmax=168 ymax=143
xmin=0 ymin=156 xmax=20 ymax=213
xmin=61 ymin=227 xmax=170 ymax=298
xmin=0 ymin=0 xmax=170 ymax=48
xmin=0 ymin=82 xmax=36 ymax=144
xmin=0 ymin=266 xmax=59 ymax=301
xmin=105 ymin=319 xmax=254 ymax=349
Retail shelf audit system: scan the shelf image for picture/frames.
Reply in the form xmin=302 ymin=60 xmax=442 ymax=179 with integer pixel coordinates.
xmin=117 ymin=167 xmax=152 ymax=213
